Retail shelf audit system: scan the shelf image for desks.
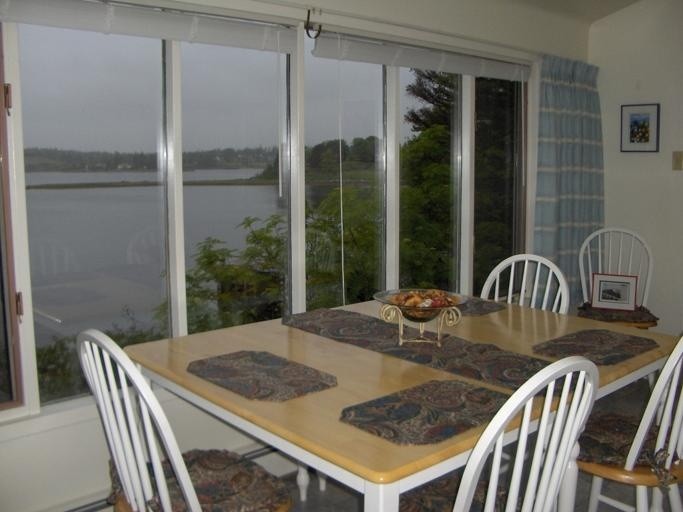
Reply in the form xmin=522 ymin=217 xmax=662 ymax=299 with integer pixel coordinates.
xmin=123 ymin=297 xmax=680 ymax=512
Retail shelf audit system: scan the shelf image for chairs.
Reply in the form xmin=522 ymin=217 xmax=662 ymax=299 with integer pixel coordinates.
xmin=480 ymin=253 xmax=570 ymax=475
xmin=577 ymin=227 xmax=659 ymax=329
xmin=76 ymin=329 xmax=293 ymax=512
xmin=578 ymin=335 xmax=683 ymax=512
xmin=400 ymin=356 xmax=600 ymax=512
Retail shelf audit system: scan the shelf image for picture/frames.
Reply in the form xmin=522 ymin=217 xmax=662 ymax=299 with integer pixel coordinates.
xmin=592 ymin=273 xmax=638 ymax=313
xmin=621 ymin=104 xmax=660 ymax=152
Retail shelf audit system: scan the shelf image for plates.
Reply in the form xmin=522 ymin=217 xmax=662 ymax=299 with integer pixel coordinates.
xmin=373 ymin=287 xmax=470 ymax=322
